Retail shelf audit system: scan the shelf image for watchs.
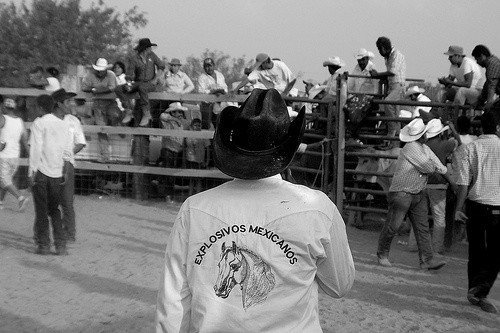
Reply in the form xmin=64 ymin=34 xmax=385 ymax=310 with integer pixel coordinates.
xmin=91 ymin=88 xmax=96 ymax=91
xmin=126 ymin=81 xmax=132 ymax=86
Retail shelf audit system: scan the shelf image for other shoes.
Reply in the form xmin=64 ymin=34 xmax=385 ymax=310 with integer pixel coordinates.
xmin=0 ymin=198 xmax=29 ymax=210
xmin=123 ymin=111 xmax=152 ymax=125
xmin=378 ymin=257 xmax=393 ymax=268
xmin=469 ymin=296 xmax=498 ymax=313
xmin=38 ymin=239 xmax=70 ymax=255
xmin=420 ymin=258 xmax=447 ymax=270
xmin=419 ymin=109 xmax=434 ymax=123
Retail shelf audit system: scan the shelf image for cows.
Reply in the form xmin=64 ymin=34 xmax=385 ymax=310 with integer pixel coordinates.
xmin=357 ymin=146 xmax=403 ymax=200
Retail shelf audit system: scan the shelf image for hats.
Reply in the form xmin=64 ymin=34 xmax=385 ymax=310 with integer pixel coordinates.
xmin=253 ymin=52 xmax=271 ymax=69
xmin=306 ymin=79 xmax=327 ymax=100
xmin=134 ymin=38 xmax=157 ymax=50
xmin=322 ymin=48 xmax=374 ymax=67
xmin=399 ymin=118 xmax=449 ymax=143
xmin=168 ymin=58 xmax=182 ymax=66
xmin=165 ymin=102 xmax=188 ymax=112
xmin=405 ymin=86 xmax=425 ymax=98
xmin=443 ymin=45 xmax=466 ymax=55
xmin=203 ymin=58 xmax=215 ymax=68
xmin=92 ymin=58 xmax=114 ymax=71
xmin=212 ymin=88 xmax=306 ymax=179
xmin=4 ymin=98 xmax=17 ymax=109
xmin=52 ymin=89 xmax=77 ymax=110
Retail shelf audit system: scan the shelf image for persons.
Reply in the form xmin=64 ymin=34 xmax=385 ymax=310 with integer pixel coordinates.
xmin=155 ymin=88 xmax=355 ymax=333
xmin=0 ymin=37 xmax=500 ymax=315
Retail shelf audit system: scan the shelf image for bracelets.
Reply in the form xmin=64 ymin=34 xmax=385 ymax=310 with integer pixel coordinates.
xmin=435 ymin=166 xmax=439 ymax=172
